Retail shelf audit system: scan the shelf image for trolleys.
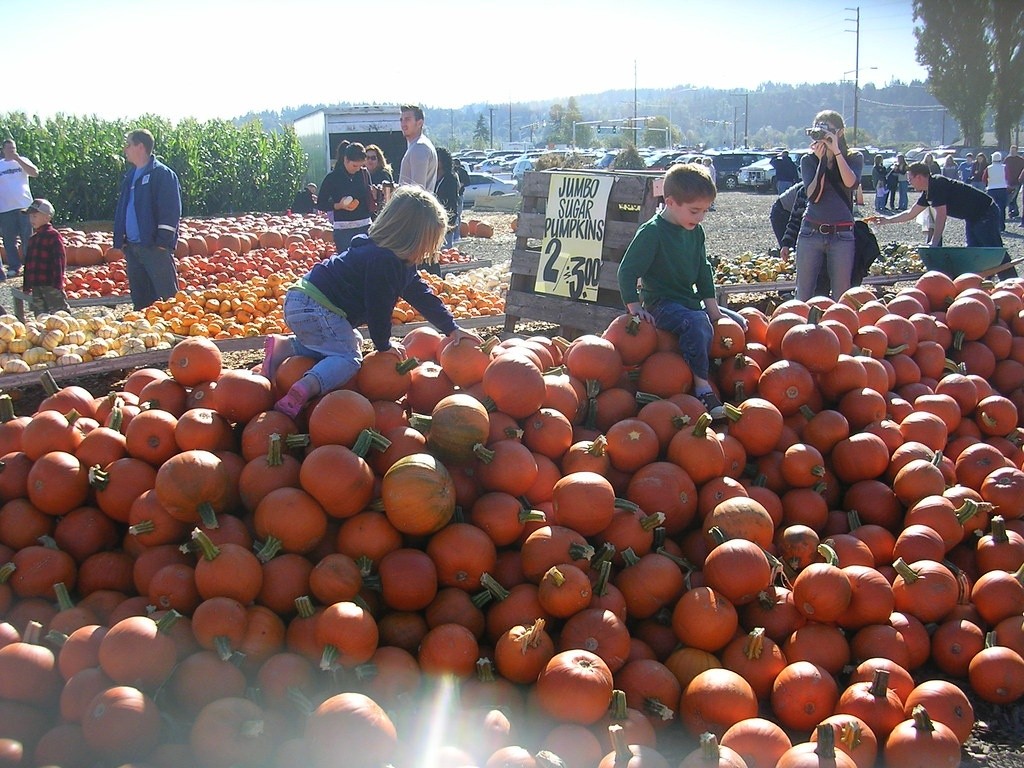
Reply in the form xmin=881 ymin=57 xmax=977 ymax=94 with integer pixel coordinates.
xmin=914 ymin=244 xmax=1024 ymax=280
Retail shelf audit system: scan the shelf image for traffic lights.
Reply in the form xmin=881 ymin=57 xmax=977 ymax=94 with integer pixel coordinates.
xmin=613 ymin=128 xmax=616 ymax=133
xmin=597 ymin=128 xmax=600 ymax=133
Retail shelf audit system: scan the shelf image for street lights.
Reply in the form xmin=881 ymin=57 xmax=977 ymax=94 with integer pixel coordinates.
xmin=668 ymin=85 xmax=697 ymax=149
xmin=842 ymin=66 xmax=880 ymax=118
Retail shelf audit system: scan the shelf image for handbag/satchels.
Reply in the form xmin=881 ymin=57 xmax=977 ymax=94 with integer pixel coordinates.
xmin=360 ymin=166 xmax=378 ymax=214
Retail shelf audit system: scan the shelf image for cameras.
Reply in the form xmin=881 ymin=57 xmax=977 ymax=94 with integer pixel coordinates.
xmin=806 ymin=123 xmax=835 ymax=143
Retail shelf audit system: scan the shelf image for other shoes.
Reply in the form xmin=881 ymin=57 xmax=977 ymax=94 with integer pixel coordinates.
xmin=6 ymin=269 xmax=17 ymax=278
xmin=259 ymin=333 xmax=292 ymax=379
xmin=273 ymin=373 xmax=320 ymax=421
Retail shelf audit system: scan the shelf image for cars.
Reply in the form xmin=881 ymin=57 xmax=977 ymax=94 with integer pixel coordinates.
xmin=463 ymin=172 xmax=516 ymax=208
xmin=708 ymin=144 xmax=1024 ymax=191
xmin=453 ymin=150 xmax=715 ymax=181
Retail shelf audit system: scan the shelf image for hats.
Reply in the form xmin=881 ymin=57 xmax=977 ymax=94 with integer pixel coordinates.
xmin=20 ymin=198 xmax=56 ymax=220
xmin=992 ymin=152 xmax=1001 ymax=161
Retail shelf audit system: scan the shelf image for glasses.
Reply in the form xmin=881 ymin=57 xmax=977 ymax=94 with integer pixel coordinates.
xmin=367 ymin=155 xmax=377 ymax=161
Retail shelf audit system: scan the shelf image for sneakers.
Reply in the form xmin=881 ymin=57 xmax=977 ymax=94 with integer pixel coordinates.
xmin=699 ymin=391 xmax=727 ymax=419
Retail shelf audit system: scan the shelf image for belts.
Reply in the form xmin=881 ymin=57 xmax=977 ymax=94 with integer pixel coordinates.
xmin=801 ymin=219 xmax=853 ymax=234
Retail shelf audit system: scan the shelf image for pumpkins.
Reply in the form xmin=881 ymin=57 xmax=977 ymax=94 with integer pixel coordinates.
xmin=0 ymin=211 xmax=1024 ymax=768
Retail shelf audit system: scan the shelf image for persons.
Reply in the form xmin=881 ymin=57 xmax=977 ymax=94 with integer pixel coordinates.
xmin=860 ymin=162 xmax=1018 ymax=282
xmin=924 ymin=145 xmax=1024 ymax=234
xmin=113 ymin=129 xmax=182 ymax=311
xmin=794 ymin=110 xmax=863 ymax=302
xmin=872 ymin=154 xmax=909 ymax=211
xmin=21 ymin=199 xmax=71 ymax=319
xmin=291 ymin=183 xmax=318 ymax=213
xmin=317 ymin=140 xmax=392 ymax=255
xmin=260 ymin=184 xmax=485 ymax=420
xmin=617 ymin=163 xmax=730 ymax=419
xmin=0 ymin=139 xmax=39 ymax=280
xmin=769 ymin=150 xmax=807 ymax=262
xmin=435 ymin=147 xmax=471 ymax=249
xmin=398 ymin=104 xmax=438 ymax=193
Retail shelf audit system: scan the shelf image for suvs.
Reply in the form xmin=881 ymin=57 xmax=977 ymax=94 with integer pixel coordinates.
xmin=714 ymin=153 xmax=769 ymax=191
